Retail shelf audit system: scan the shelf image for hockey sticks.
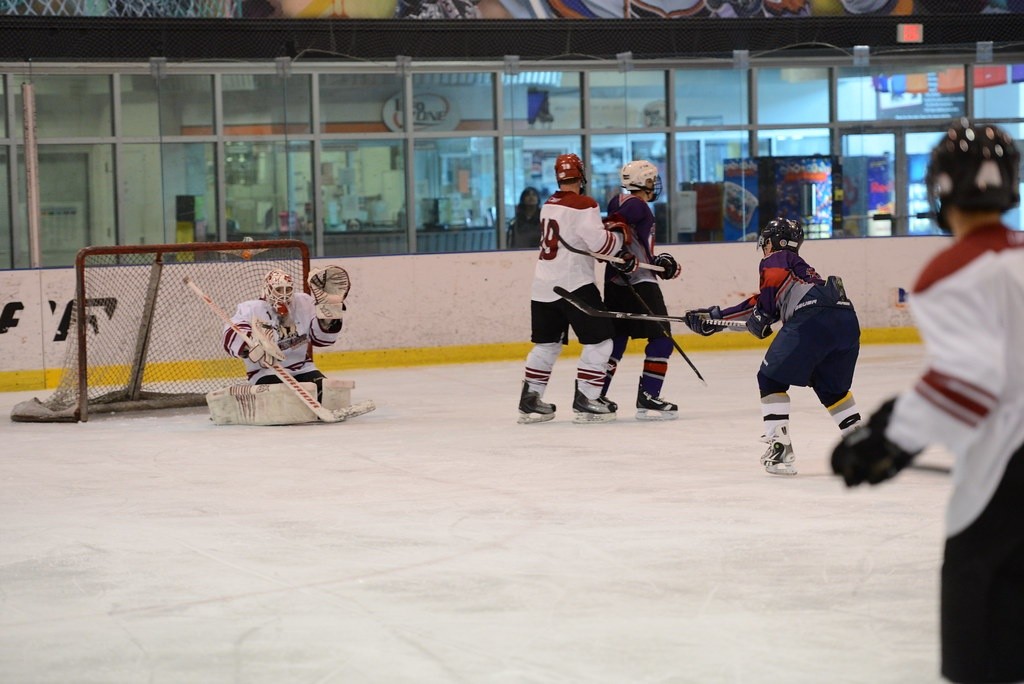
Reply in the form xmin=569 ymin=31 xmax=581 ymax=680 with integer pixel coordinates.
xmin=587 ymin=250 xmax=665 ymax=273
xmin=553 ymin=286 xmax=748 ymax=329
xmin=612 ymin=263 xmax=704 ymax=382
xmin=183 ymin=277 xmax=375 ymax=421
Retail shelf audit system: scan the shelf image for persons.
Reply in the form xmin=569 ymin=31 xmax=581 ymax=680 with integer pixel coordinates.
xmin=219 ymin=267 xmax=352 ymax=420
xmin=346 ymin=218 xmax=361 ymax=231
xmin=599 ymin=159 xmax=682 ymax=422
xmin=511 ymin=186 xmax=542 ymax=248
xmin=830 ymin=114 xmax=1024 ymax=684
xmin=684 ymin=215 xmax=861 ymax=474
xmin=516 ymin=152 xmax=633 ymax=423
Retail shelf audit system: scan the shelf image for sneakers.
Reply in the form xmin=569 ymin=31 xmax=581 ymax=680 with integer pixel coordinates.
xmin=634 ymin=375 xmax=679 ymax=422
xmin=514 ymin=381 xmax=558 ymax=424
xmin=758 ymin=423 xmax=798 ymax=476
xmin=258 ymin=267 xmax=294 ymax=311
xmin=569 ymin=378 xmax=619 ymax=424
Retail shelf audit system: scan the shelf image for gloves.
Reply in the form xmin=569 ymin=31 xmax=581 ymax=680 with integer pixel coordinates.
xmin=657 ymin=253 xmax=678 ymax=279
xmin=611 ymin=250 xmax=640 ymax=275
xmin=744 ymin=307 xmax=775 ymax=339
xmin=679 ymin=305 xmax=726 ymax=336
xmin=829 ymin=396 xmax=922 ymax=488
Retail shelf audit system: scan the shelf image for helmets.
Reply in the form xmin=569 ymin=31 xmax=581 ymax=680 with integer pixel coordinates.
xmin=924 ymin=116 xmax=1021 ymax=227
xmin=755 ymin=216 xmax=805 ymax=255
xmin=617 ymin=160 xmax=662 ymax=204
xmin=554 ymin=153 xmax=588 ymax=192
